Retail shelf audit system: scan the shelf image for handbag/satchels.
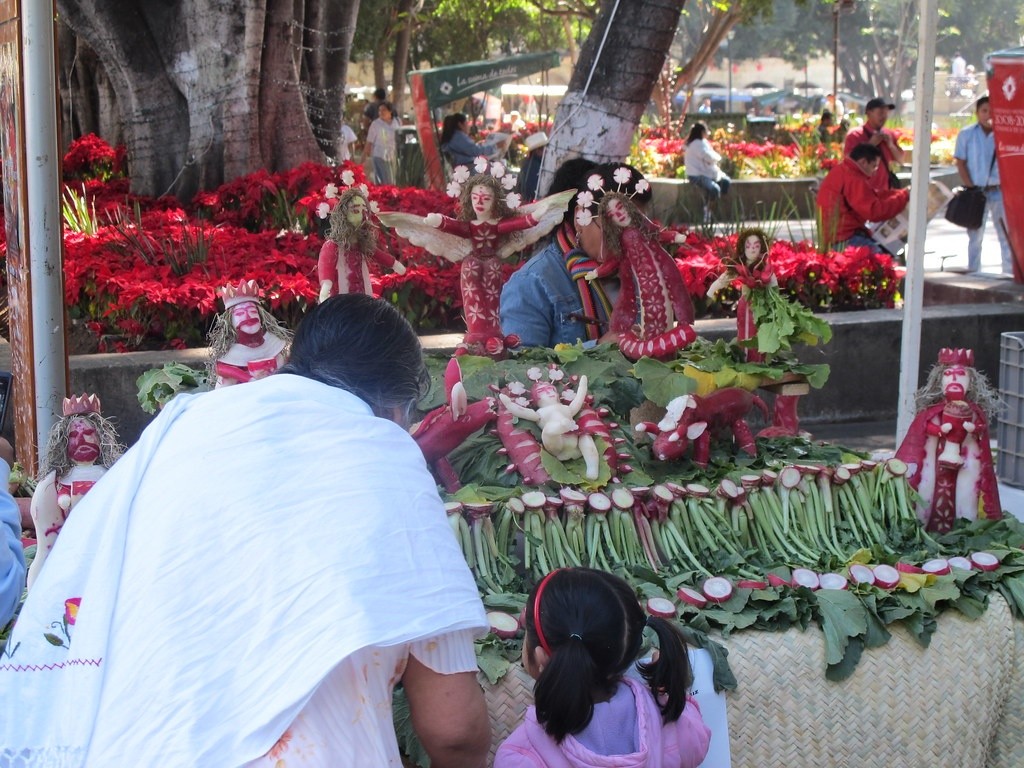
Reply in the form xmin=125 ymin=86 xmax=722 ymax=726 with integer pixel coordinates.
xmin=946 ymin=191 xmax=985 ymax=228
xmin=888 ymin=170 xmax=900 ymax=189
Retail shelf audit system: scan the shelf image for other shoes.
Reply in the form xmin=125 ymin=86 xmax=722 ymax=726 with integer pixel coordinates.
xmin=704 ymin=205 xmax=712 ymax=224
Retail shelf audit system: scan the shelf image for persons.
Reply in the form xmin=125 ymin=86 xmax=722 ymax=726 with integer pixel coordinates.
xmin=817 ymin=142 xmax=911 ymax=255
xmin=341 ymin=87 xmax=403 ymax=187
xmin=584 ymin=192 xmax=697 ymax=359
xmin=499 ymin=374 xmax=599 ymax=480
xmin=953 ymin=96 xmax=1013 ymax=273
xmin=440 ymin=113 xmax=506 ymax=174
xmin=706 ymin=227 xmax=779 ymax=363
xmin=894 ymin=347 xmax=1004 ymax=531
xmin=518 ymin=95 xmax=538 ymax=114
xmin=0 ymin=370 xmax=34 ymax=633
xmin=0 ymin=291 xmax=492 ymax=768
xmin=844 ymin=98 xmax=906 ymax=189
xmin=699 ymin=96 xmax=711 ymax=113
xmin=318 ymin=190 xmax=405 ymax=302
xmin=27 ymin=392 xmax=126 ymax=592
xmin=208 ymin=279 xmax=296 ymax=390
xmin=500 ymin=152 xmax=652 ymax=348
xmin=423 ymin=173 xmax=550 ymax=343
xmin=492 ymin=564 xmax=711 ymax=768
xmin=685 ymin=120 xmax=731 ymax=222
xmin=813 ymin=93 xmax=850 ymax=142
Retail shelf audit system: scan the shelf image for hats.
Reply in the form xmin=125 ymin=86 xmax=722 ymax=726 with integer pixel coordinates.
xmin=867 ymin=98 xmax=895 ymax=111
xmin=525 ymin=132 xmax=548 ymax=152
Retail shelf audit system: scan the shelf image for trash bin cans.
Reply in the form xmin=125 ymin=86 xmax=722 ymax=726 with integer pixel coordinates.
xmin=746 ymin=116 xmax=779 ymax=144
xmin=394 ymin=125 xmax=420 ymax=166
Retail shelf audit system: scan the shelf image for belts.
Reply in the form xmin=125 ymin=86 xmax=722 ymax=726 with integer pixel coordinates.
xmin=985 ymin=185 xmax=1001 ymax=192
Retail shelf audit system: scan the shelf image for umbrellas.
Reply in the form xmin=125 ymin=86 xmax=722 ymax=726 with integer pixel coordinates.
xmin=698 ymin=82 xmax=818 ymax=96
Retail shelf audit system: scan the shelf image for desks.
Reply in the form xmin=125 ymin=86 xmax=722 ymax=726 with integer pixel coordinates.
xmin=478 ymin=587 xmax=1024 ymax=768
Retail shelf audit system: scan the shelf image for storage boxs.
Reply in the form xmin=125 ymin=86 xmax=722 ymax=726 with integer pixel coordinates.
xmin=996 ymin=332 xmax=1024 ymax=492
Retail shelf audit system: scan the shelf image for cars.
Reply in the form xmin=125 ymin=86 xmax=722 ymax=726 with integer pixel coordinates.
xmin=645 ymin=85 xmax=875 ymax=118
